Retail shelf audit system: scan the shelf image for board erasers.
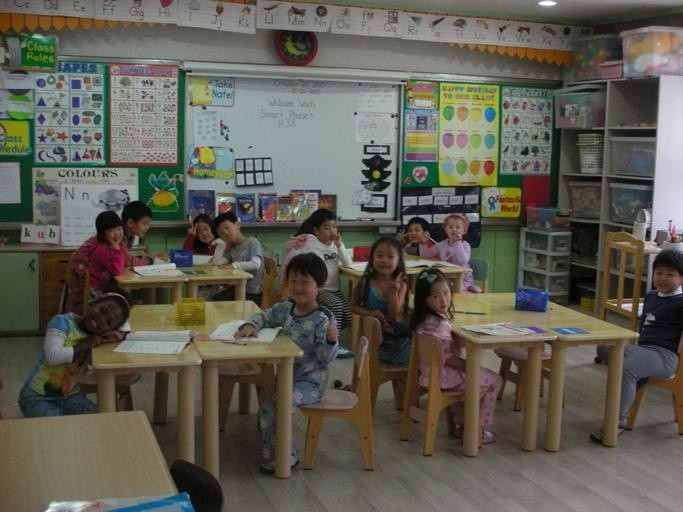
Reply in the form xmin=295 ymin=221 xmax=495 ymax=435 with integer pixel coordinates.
xmin=340 ymin=216 xmax=358 ymax=221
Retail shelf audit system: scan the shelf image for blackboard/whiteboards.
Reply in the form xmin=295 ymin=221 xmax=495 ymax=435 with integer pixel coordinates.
xmin=402 ymin=79 xmax=558 ymax=224
xmin=1 ymin=58 xmax=185 ymax=229
xmin=183 ymin=75 xmax=402 ymax=226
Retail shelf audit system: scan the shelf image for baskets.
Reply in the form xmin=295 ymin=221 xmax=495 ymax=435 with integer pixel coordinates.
xmin=515 ymin=287 xmax=548 ymax=312
xmin=178 ymin=298 xmax=205 ymax=325
xmin=578 ymin=133 xmax=604 ymax=174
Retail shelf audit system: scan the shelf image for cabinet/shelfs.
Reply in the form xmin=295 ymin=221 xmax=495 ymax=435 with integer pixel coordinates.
xmin=514 ymin=227 xmax=573 ymax=304
xmin=556 ymin=77 xmax=682 ymax=312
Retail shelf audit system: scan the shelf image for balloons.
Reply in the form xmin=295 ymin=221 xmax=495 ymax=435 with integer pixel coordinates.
xmin=442 ymin=105 xmax=496 ymax=179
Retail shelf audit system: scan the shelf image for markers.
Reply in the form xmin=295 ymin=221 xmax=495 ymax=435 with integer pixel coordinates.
xmin=360 ymin=219 xmax=375 ymax=221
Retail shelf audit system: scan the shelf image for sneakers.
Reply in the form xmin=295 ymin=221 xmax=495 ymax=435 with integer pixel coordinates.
xmin=480 ymin=429 xmax=496 ymax=444
xmin=260 ymin=454 xmax=300 ymax=474
xmin=337 ymin=345 xmax=352 ymax=358
xmin=591 ymin=426 xmax=624 ymax=442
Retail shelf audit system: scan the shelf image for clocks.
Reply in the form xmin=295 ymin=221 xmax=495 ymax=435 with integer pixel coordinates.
xmin=273 ymin=30 xmax=318 ymax=66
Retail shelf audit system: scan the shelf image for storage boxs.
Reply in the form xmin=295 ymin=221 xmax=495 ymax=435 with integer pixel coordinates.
xmin=522 ymin=205 xmax=572 ymax=231
xmin=610 ymin=136 xmax=657 ymax=176
xmin=565 ymin=177 xmax=601 ymax=219
xmin=570 ymin=36 xmax=622 ymax=78
xmin=620 ymin=23 xmax=683 ymax=74
xmin=554 ymin=82 xmax=605 ymax=133
xmin=610 ymin=180 xmax=654 ymax=223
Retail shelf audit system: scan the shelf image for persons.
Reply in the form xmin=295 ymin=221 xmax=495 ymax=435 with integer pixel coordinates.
xmin=19 ymin=288 xmax=132 ymax=416
xmin=588 ymin=251 xmax=682 ymax=443
xmin=397 ymin=215 xmax=473 ymax=293
xmin=347 ymin=237 xmax=411 ymax=367
xmin=182 ymin=212 xmax=263 ymax=309
xmin=71 ymin=201 xmax=169 ymax=309
xmin=235 ymin=253 xmax=338 ymax=475
xmin=410 ymin=269 xmax=502 ymax=444
xmin=280 ymin=213 xmax=354 ymax=356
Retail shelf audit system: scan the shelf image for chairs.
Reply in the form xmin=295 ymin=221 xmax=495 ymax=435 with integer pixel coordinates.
xmin=1 ymin=231 xmax=681 ymax=512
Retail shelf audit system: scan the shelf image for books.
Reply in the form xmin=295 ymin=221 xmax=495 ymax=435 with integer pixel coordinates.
xmin=46 ymin=491 xmax=194 ymax=511
xmin=186 ymin=187 xmax=335 ymax=222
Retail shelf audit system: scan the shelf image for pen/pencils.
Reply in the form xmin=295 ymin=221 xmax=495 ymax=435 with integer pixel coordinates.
xmin=429 ymin=237 xmax=439 ymax=245
xmin=224 ymin=342 xmax=247 ymax=345
xmin=668 ymin=220 xmax=681 ymax=242
xmin=456 ymin=311 xmax=485 ymax=315
